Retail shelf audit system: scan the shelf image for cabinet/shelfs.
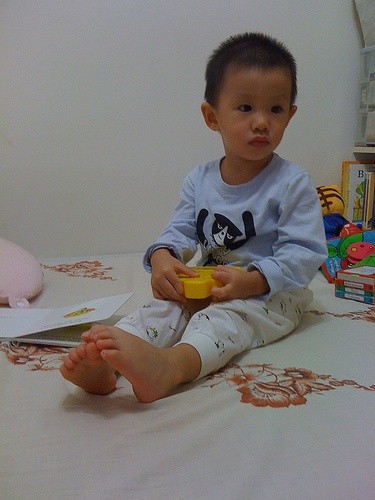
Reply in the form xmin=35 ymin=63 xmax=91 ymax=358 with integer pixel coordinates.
xmin=352 ymin=45 xmax=375 ymax=153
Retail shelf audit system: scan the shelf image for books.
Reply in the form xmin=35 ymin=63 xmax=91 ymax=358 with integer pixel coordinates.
xmin=320 ymin=160 xmax=375 ymax=305
xmin=0 ymin=291 xmax=134 ymax=348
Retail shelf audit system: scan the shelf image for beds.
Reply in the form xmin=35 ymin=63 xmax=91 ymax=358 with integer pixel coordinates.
xmin=0 ymin=252 xmax=375 ymax=500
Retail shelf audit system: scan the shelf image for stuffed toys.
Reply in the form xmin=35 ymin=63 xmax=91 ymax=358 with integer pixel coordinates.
xmin=314 ymin=184 xmax=362 ymax=239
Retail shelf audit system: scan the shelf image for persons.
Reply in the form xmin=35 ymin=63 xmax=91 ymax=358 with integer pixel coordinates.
xmin=57 ymin=33 xmax=328 ymax=403
